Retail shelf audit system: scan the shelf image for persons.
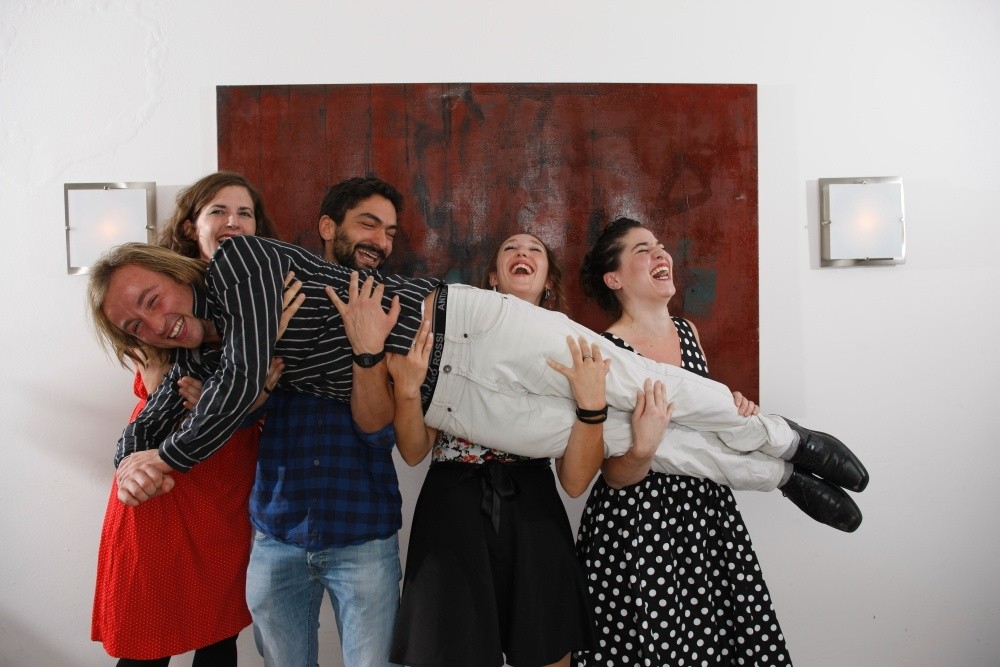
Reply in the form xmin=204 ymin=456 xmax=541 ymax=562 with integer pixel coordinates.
xmin=572 ymin=219 xmax=795 ymax=667
xmin=235 ymin=174 xmax=402 ymax=667
xmin=382 ymin=233 xmax=612 ymax=667
xmin=87 ymin=236 xmax=871 ymax=532
xmin=89 ymin=171 xmax=305 ymax=667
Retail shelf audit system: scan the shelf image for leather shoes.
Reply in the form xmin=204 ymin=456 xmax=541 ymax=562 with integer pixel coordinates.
xmin=778 ymin=463 xmax=862 ymax=533
xmin=775 ymin=414 xmax=869 ymax=493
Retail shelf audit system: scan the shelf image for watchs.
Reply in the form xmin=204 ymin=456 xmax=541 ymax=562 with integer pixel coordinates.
xmin=353 ymin=344 xmax=386 ymax=369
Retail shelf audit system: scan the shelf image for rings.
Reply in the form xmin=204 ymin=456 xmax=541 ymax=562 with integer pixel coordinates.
xmin=583 ymin=355 xmax=594 ymax=362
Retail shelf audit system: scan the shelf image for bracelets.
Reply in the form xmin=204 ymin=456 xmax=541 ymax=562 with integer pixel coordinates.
xmin=574 ymin=403 xmax=608 ymax=424
